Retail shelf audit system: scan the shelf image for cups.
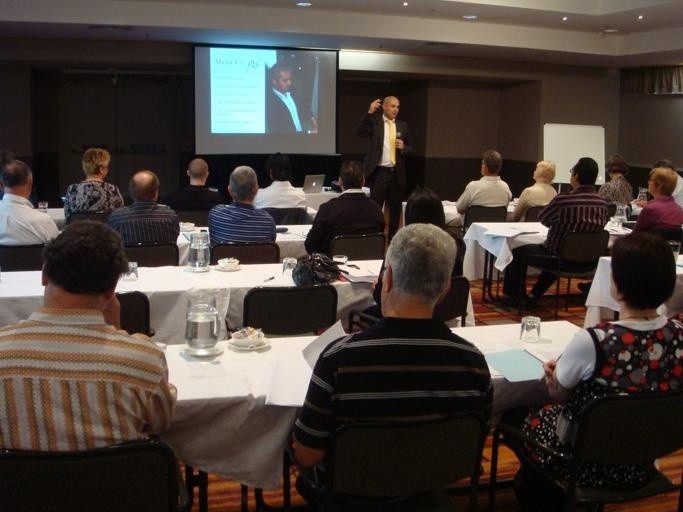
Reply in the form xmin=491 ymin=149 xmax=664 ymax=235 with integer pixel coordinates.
xmin=520 ymin=315 xmax=541 ymax=342
xmin=332 ymin=255 xmax=347 ymax=268
xmin=124 ymin=261 xmax=138 ymax=281
xmin=670 ymin=240 xmax=682 ymax=266
xmin=282 ymin=257 xmax=296 ymax=279
xmin=37 ymin=202 xmax=47 ymax=212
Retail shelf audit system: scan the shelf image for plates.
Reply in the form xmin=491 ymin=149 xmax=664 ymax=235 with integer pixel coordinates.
xmin=226 ymin=340 xmax=269 ymax=350
xmin=215 ymin=266 xmax=240 ymax=271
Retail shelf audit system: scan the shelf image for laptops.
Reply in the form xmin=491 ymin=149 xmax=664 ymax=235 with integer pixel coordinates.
xmin=302 ymin=174 xmax=325 ymax=194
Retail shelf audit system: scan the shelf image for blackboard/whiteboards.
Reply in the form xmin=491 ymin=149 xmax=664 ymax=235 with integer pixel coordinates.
xmin=543 ymin=123 xmax=605 ymax=185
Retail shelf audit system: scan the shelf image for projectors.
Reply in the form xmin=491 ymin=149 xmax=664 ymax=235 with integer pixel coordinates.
xmin=331 ymin=179 xmax=342 ymax=193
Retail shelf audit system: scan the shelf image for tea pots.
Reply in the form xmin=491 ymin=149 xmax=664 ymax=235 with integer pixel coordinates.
xmin=183 ymin=287 xmax=222 ymax=356
xmin=188 ymin=232 xmax=210 ymax=274
xmin=614 ymin=201 xmax=631 ymax=226
xmin=637 ymin=187 xmax=651 ymax=202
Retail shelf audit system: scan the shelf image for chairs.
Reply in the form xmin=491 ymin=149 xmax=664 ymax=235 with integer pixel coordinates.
xmin=0 ymin=186 xmax=683 ymax=512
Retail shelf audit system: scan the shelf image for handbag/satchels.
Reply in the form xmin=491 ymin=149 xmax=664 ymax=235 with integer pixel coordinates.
xmin=292 ymin=253 xmax=345 ymax=288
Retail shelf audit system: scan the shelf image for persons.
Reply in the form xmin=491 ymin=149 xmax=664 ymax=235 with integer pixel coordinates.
xmin=355 ymin=95 xmax=410 ymax=245
xmin=266 ymin=63 xmax=318 ymax=135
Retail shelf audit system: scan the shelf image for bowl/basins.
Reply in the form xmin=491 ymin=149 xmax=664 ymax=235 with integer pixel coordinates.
xmin=217 ymin=260 xmax=239 ymax=269
xmin=231 ymin=333 xmax=264 ymax=347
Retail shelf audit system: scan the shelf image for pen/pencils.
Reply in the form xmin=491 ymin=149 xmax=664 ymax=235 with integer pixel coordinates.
xmin=555 ymin=352 xmax=563 ymax=362
xmin=264 ymin=276 xmax=275 ymax=283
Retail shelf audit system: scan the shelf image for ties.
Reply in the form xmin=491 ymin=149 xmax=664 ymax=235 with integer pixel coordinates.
xmin=386 ymin=119 xmax=397 ymax=166
